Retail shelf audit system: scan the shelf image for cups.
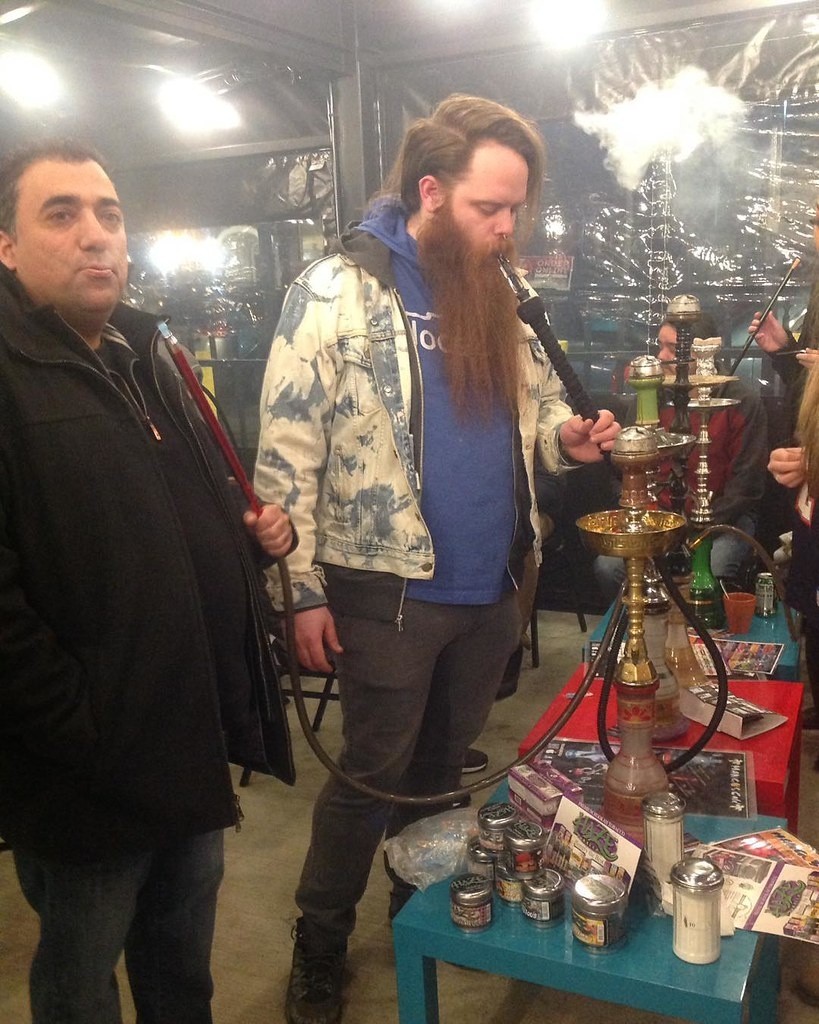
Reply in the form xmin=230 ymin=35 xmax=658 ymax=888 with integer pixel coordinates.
xmin=724 ymin=592 xmax=757 ymax=634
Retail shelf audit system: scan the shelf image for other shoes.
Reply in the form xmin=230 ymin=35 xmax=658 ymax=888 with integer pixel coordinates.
xmin=496 ymin=646 xmax=523 ymax=702
xmin=801 ymin=707 xmax=819 ymax=729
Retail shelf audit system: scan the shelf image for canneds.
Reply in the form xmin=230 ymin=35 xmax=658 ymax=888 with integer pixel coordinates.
xmin=444 ymin=800 xmax=629 ymax=954
xmin=754 ymin=572 xmax=777 ymax=618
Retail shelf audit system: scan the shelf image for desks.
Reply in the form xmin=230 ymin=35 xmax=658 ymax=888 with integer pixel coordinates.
xmin=391 ymin=579 xmax=806 ymax=1024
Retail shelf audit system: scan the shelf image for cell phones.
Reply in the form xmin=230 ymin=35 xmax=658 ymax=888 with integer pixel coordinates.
xmin=777 ymin=350 xmax=806 ymax=366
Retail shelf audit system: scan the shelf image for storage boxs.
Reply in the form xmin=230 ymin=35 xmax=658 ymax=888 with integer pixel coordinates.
xmin=503 ymin=762 xmax=584 ymax=829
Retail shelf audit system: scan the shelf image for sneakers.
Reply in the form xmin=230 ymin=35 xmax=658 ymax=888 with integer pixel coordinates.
xmin=284 ymin=911 xmax=347 ymax=1024
xmin=452 ymin=786 xmax=471 ymax=808
xmin=387 ymin=878 xmax=417 ymax=920
xmin=462 ymin=748 xmax=488 ymax=772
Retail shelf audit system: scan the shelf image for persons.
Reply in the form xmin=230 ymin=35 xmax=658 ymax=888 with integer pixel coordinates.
xmin=748 ymin=210 xmax=819 ymax=771
xmin=0 ymin=144 xmax=299 ymax=1024
xmin=594 ymin=311 xmax=767 ymax=613
xmin=252 ymin=93 xmax=621 ymax=1023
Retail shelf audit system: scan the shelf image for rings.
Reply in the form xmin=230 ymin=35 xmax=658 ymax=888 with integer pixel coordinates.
xmin=303 ymin=661 xmax=312 ymax=667
xmin=775 ymin=473 xmax=781 ymax=484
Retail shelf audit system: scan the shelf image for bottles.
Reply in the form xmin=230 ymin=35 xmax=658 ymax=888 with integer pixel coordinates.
xmin=640 ymin=792 xmax=686 ymax=916
xmin=670 ymin=858 xmax=725 ymax=965
